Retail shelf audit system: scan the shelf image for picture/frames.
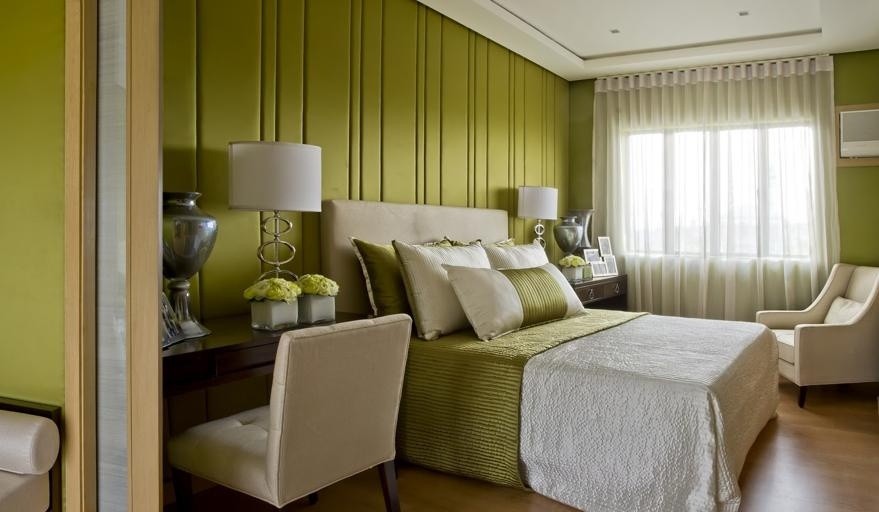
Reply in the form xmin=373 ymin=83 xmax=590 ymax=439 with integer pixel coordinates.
xmin=590 ymin=261 xmax=609 ymax=277
xmin=604 ymin=256 xmax=618 ymax=275
xmin=582 ymin=248 xmax=602 ymax=264
xmin=598 ymin=236 xmax=613 ymax=256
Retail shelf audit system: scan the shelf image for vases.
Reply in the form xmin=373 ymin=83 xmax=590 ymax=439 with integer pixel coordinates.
xmin=562 ymin=267 xmax=583 ymax=282
xmin=298 ymin=295 xmax=336 ymax=325
xmin=251 ymin=300 xmax=298 ymax=331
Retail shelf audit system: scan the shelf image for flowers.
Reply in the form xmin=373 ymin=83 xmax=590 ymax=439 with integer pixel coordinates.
xmin=558 ymin=254 xmax=586 ymax=267
xmin=295 ymin=274 xmax=340 ymax=297
xmin=243 ymin=278 xmax=303 ymax=305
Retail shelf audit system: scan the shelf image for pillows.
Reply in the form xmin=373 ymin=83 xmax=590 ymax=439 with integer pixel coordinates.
xmin=441 ymin=263 xmax=587 ymax=343
xmin=390 ymin=238 xmax=493 ymax=342
xmin=479 ymin=240 xmax=550 ymax=270
xmin=348 ymin=235 xmax=453 ymax=317
xmin=447 ymin=237 xmax=515 ymax=249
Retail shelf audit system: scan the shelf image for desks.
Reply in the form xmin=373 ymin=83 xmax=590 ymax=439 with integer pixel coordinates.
xmin=163 ymin=312 xmax=373 ymax=438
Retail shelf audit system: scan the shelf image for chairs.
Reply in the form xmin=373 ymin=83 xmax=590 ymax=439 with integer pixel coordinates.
xmin=160 ymin=312 xmax=414 ymax=512
xmin=756 ymin=262 xmax=878 ymax=408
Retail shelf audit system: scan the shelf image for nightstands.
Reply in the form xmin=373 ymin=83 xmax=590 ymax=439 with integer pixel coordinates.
xmin=571 ymin=275 xmax=628 ymax=311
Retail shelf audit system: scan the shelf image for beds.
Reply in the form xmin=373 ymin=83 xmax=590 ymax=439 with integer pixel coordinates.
xmin=318 ymin=200 xmax=783 ymax=512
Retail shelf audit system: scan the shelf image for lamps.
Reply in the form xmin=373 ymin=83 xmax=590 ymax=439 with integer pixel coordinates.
xmin=228 ymin=140 xmax=322 ymax=281
xmin=517 ymin=186 xmax=558 ymax=249
